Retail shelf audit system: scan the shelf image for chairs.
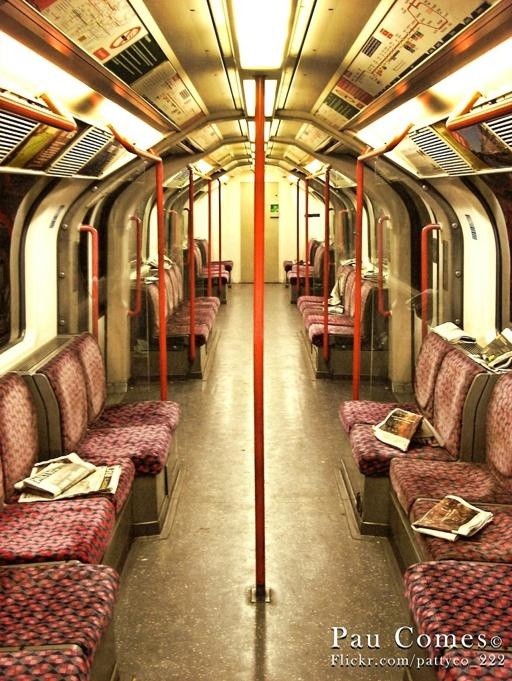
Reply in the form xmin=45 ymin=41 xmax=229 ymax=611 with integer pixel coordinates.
xmin=0 ymin=239 xmax=511 ymax=681
